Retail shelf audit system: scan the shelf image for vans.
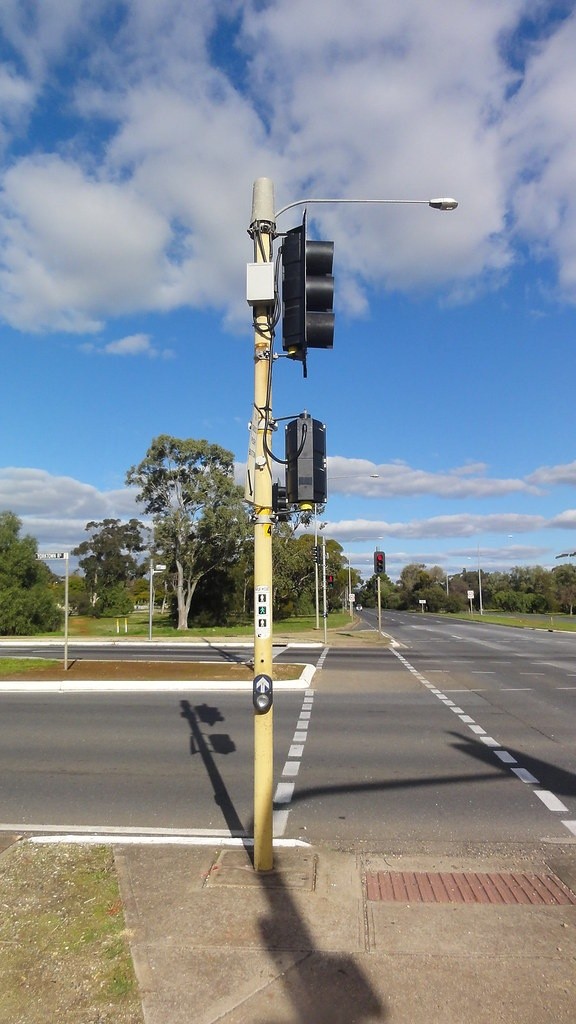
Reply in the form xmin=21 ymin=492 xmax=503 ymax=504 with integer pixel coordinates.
xmin=355 ymin=604 xmax=362 ymax=611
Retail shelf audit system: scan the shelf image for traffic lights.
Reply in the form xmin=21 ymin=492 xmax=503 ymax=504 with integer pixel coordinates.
xmin=374 ymin=551 xmax=385 ymax=573
xmin=282 ymin=207 xmax=335 ymax=376
xmin=327 ymin=576 xmax=333 ymax=586
xmin=271 ymin=483 xmax=290 ymax=522
xmin=313 ymin=546 xmax=321 ymax=564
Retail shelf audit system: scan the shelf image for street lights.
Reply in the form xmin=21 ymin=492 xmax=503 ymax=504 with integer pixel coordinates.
xmin=348 ymin=536 xmax=382 ymax=617
xmin=446 ymin=557 xmax=471 ymax=596
xmin=314 ymin=475 xmax=379 ymax=629
xmin=477 ymin=534 xmax=513 ymax=615
xmin=252 ymin=198 xmax=458 ymax=871
